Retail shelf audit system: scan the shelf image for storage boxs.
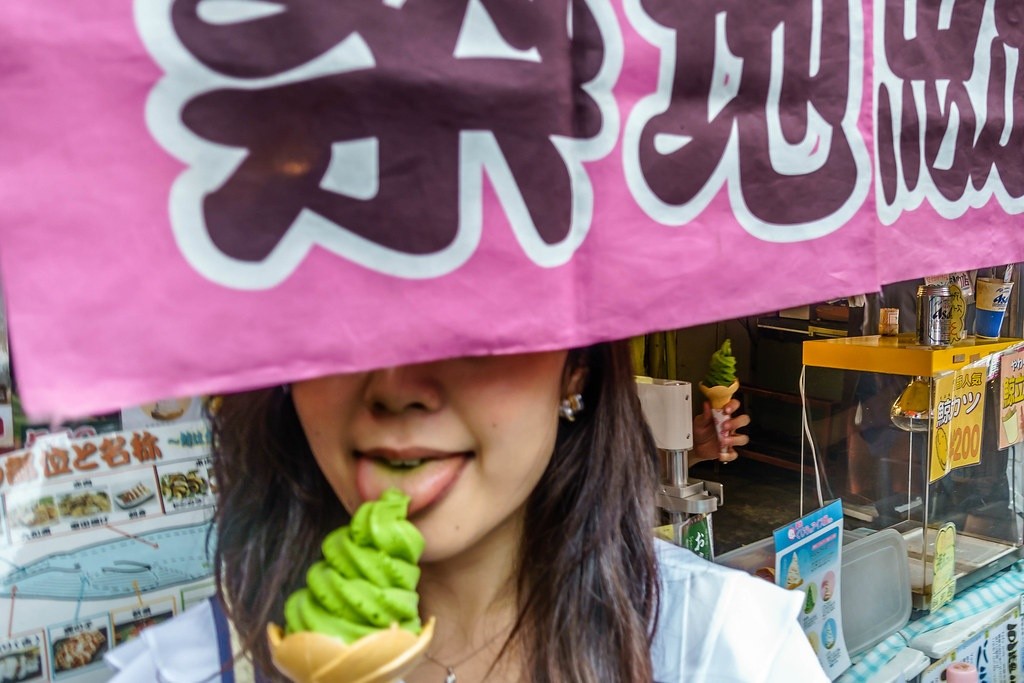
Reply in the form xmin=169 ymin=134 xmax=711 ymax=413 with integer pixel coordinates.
xmin=713 ymin=528 xmax=914 ymax=658
xmin=800 ymin=331 xmax=1024 ymax=611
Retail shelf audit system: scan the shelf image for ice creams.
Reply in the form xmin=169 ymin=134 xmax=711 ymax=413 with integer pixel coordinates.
xmin=698 ymin=339 xmax=739 ymax=464
xmin=266 ymin=487 xmax=435 ymax=683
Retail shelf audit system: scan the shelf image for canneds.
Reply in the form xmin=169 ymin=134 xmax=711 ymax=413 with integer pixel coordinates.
xmin=917 ymin=285 xmax=952 ymax=350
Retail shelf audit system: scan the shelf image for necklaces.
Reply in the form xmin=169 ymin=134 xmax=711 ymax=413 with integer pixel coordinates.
xmin=424 ymin=615 xmax=512 ymax=683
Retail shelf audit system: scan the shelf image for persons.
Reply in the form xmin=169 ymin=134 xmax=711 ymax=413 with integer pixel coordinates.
xmin=688 ymin=397 xmax=751 ymax=469
xmin=97 ymin=338 xmax=834 ymax=683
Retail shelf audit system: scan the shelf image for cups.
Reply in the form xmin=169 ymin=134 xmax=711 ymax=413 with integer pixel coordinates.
xmin=974 ymin=276 xmax=1015 ymax=339
